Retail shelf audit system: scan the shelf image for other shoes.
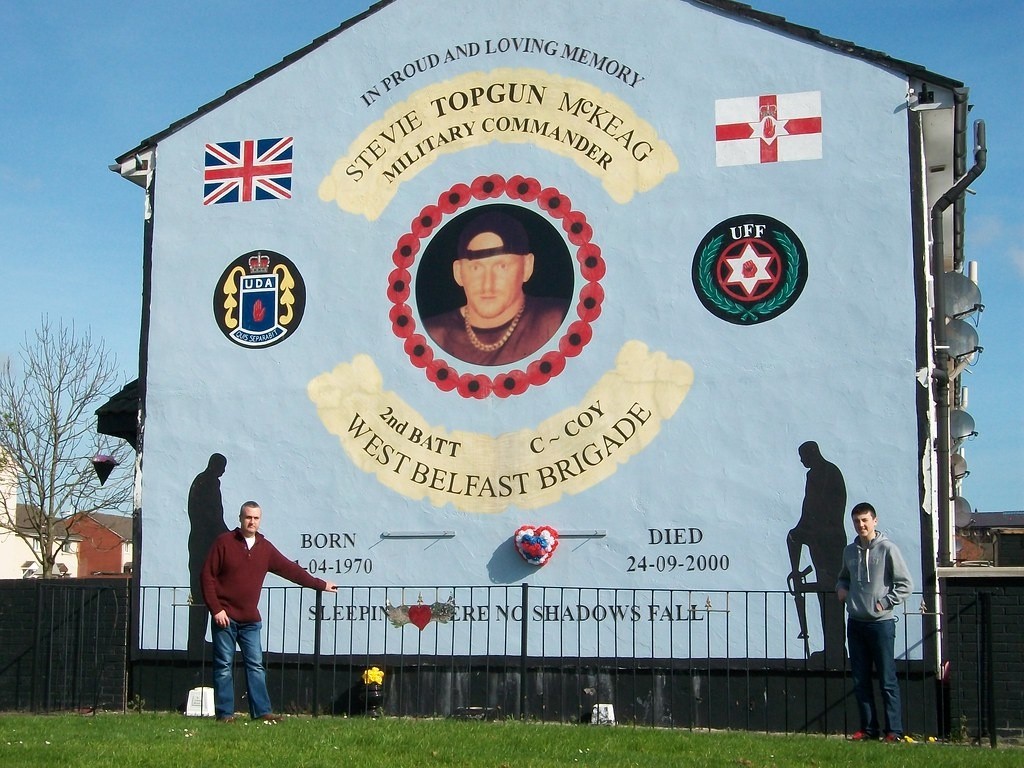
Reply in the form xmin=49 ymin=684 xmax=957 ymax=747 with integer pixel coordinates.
xmin=258 ymin=714 xmax=283 ymax=721
xmin=216 ymin=716 xmax=235 ymax=723
xmin=881 ymin=733 xmax=901 ymax=742
xmin=852 ymin=731 xmax=872 ymax=740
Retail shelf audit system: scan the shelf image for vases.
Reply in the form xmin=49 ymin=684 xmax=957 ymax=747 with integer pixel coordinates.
xmin=362 ymin=682 xmax=386 ymax=717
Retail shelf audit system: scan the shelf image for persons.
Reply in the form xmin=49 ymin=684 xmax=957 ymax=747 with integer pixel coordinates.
xmin=836 ymin=502 xmax=914 ymax=744
xmin=200 ymin=501 xmax=337 ymax=722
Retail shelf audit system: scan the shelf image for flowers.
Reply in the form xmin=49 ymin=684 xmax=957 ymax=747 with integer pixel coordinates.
xmin=360 ymin=666 xmax=386 ymax=687
